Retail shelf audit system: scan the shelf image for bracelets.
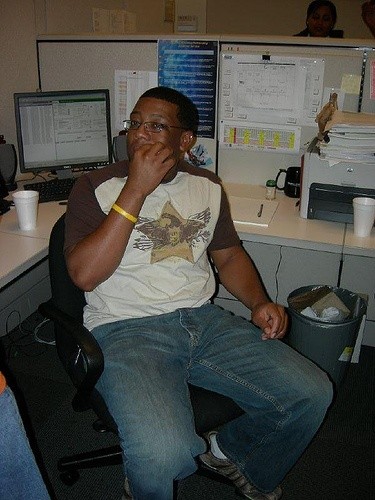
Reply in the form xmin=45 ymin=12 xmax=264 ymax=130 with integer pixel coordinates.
xmin=112 ymin=204 xmax=137 ymax=223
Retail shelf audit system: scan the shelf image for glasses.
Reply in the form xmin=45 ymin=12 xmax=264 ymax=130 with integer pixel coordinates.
xmin=122 ymin=119 xmax=190 ymax=135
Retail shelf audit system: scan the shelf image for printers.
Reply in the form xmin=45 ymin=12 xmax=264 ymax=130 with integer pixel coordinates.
xmin=300 ymin=152 xmax=375 ymax=227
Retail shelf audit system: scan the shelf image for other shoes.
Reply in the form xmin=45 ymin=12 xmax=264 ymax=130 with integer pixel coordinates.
xmin=195 ymin=431 xmax=283 ymax=500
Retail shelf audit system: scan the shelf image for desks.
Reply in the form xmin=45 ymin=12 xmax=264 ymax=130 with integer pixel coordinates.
xmin=37 ymin=210 xmax=246 ymax=500
xmin=0 ymin=172 xmax=68 ymax=339
xmin=217 ymin=182 xmax=375 ymax=258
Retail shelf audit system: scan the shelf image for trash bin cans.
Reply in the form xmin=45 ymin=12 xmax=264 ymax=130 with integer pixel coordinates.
xmin=288 ymin=285 xmax=367 ymax=384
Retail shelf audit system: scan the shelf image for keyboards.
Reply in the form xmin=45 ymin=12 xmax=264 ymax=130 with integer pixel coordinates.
xmin=23 ymin=179 xmax=78 ymax=204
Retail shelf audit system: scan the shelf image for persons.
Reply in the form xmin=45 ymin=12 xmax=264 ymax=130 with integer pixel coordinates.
xmin=292 ymin=0 xmax=344 ymax=39
xmin=0 ymin=372 xmax=51 ymax=500
xmin=63 ymin=86 xmax=332 ymax=500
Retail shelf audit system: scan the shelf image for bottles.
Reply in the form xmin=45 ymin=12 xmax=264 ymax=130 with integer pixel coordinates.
xmin=265 ymin=179 xmax=276 ymax=200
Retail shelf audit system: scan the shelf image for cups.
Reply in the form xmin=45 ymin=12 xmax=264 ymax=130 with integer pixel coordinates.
xmin=352 ymin=196 xmax=375 ymax=238
xmin=275 ymin=165 xmax=302 ymax=198
xmin=12 ymin=190 xmax=40 ymax=231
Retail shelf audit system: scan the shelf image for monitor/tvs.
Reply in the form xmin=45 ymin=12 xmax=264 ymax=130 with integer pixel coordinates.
xmin=14 ymin=89 xmax=113 ymax=178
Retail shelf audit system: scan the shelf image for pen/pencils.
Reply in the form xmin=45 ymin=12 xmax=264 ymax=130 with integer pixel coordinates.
xmin=257 ymin=204 xmax=263 ymax=217
xmin=59 ymin=202 xmax=67 ymax=205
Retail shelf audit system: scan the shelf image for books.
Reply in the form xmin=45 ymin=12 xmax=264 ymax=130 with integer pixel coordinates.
xmin=227 ymin=196 xmax=279 ymax=227
xmin=319 ymin=110 xmax=375 ymax=162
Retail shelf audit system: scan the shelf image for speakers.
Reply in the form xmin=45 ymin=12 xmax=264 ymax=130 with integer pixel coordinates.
xmin=0 ymin=143 xmax=17 ymax=191
xmin=113 ymin=135 xmax=129 ymax=162
